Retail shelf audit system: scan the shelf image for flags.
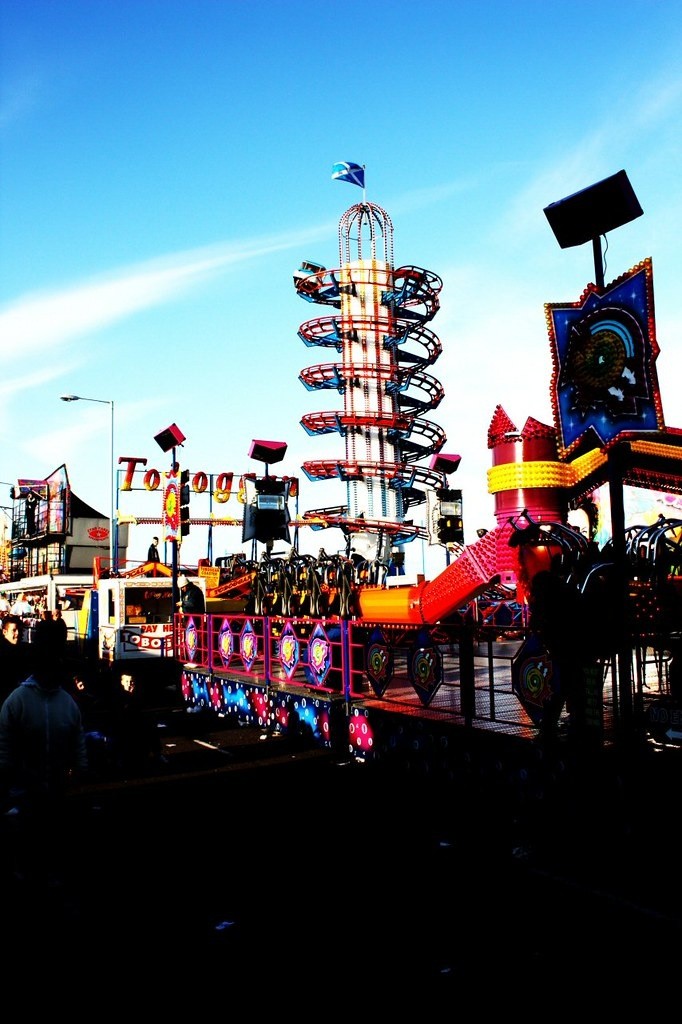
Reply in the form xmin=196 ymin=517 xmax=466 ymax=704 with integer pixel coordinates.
xmin=331 ymin=162 xmax=364 ymax=188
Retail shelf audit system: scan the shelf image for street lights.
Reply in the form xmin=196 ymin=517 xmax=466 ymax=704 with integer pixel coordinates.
xmin=60 ymin=394 xmax=116 ymax=572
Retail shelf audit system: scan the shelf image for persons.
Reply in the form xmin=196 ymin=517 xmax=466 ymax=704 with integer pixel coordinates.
xmin=0 ymin=608 xmax=87 ymax=823
xmin=148 ymin=537 xmax=160 ymax=576
xmin=175 ymin=576 xmax=205 ymax=628
